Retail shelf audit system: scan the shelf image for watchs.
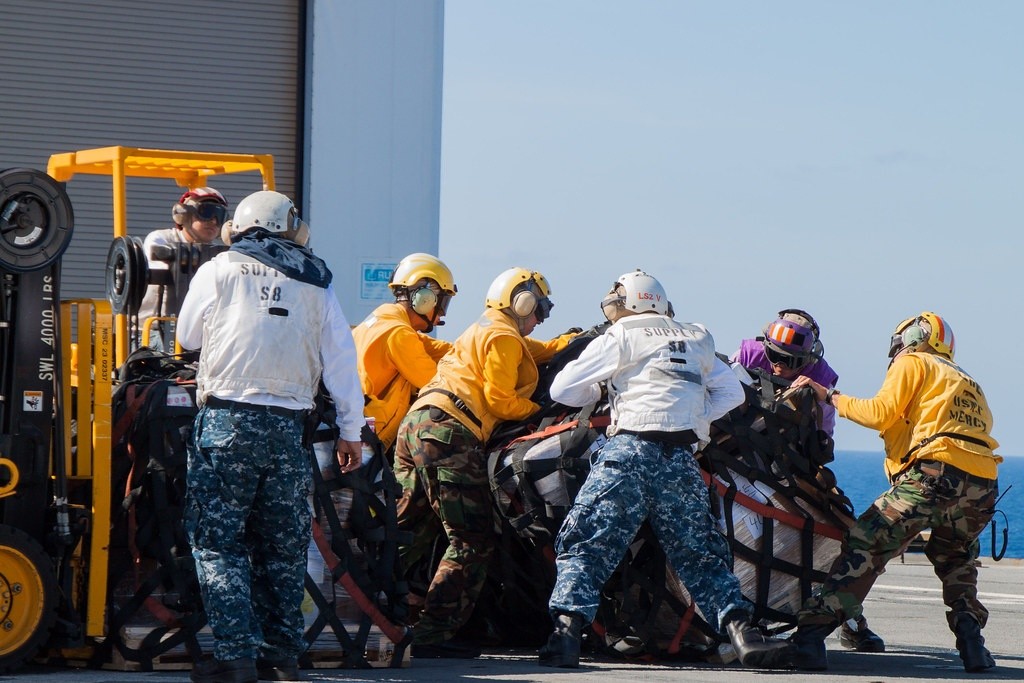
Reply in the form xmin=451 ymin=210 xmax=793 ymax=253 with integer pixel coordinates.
xmin=825 ymin=388 xmax=840 ymax=405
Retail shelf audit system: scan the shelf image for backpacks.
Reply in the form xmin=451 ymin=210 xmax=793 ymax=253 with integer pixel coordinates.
xmin=114 ymin=345 xmax=203 ymax=625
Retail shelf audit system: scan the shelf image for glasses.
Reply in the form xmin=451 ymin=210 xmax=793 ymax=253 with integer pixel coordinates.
xmin=436 ymin=292 xmax=452 ymax=315
xmin=534 ymin=298 xmax=555 ymax=323
xmin=765 ymin=345 xmax=809 ymax=370
xmin=197 ymin=202 xmax=230 ymax=226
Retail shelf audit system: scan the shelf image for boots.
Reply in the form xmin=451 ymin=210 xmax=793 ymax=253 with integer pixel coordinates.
xmin=956 ymin=613 xmax=997 ymax=672
xmin=537 ymin=611 xmax=584 ymax=668
xmin=723 ymin=607 xmax=799 ymax=668
xmin=774 ymin=608 xmax=842 ymax=670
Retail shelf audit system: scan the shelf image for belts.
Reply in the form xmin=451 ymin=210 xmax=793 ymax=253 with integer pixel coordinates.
xmin=918 ymin=458 xmax=997 ymax=489
xmin=205 ymin=394 xmax=310 ymax=424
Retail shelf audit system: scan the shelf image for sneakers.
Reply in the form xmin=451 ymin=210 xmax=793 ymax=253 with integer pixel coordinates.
xmin=841 ymin=614 xmax=885 ymax=652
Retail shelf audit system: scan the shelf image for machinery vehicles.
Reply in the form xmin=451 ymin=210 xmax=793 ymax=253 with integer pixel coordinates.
xmin=0 ymin=144 xmax=276 ymax=675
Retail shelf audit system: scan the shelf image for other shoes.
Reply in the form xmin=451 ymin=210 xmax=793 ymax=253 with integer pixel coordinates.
xmin=255 ymin=650 xmax=299 ymax=680
xmin=190 ymin=656 xmax=259 ymax=682
xmin=410 ymin=637 xmax=482 ymax=658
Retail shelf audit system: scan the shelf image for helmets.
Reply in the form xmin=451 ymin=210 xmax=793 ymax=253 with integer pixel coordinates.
xmin=609 ymin=272 xmax=668 ymax=314
xmin=485 ymin=268 xmax=552 ymax=307
xmin=233 ymin=190 xmax=299 ymax=234
xmin=175 ymin=187 xmax=229 ymax=231
xmin=888 ymin=312 xmax=956 ymax=362
xmin=762 ymin=308 xmax=824 ymax=379
xmin=389 ymin=253 xmax=458 ymax=296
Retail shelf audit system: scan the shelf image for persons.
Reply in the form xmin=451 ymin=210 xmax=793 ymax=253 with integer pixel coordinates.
xmin=175 ymin=190 xmax=365 ymax=683
xmin=786 ymin=312 xmax=1003 ymax=673
xmin=306 ymin=252 xmax=885 ymax=667
xmin=140 ymin=184 xmax=229 ymax=351
